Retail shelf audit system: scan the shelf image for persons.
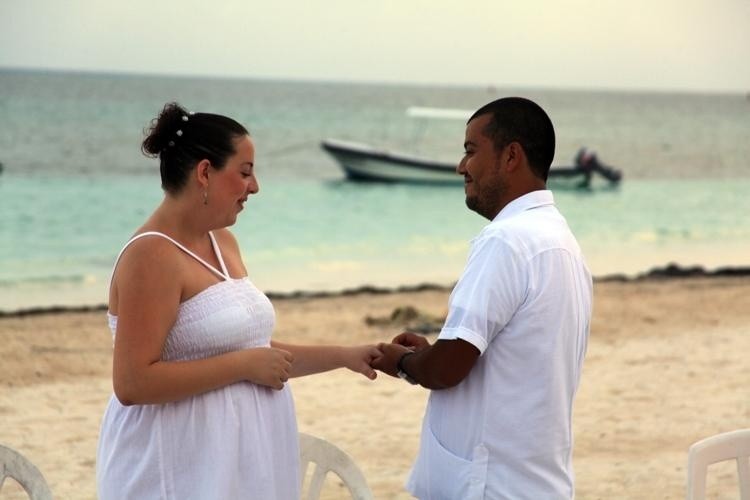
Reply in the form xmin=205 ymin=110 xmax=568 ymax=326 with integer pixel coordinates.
xmin=95 ymin=102 xmax=417 ymax=499
xmin=371 ymin=97 xmax=597 ymax=500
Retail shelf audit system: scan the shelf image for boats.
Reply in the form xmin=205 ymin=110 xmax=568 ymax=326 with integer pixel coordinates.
xmin=320 ymin=136 xmax=620 ymax=191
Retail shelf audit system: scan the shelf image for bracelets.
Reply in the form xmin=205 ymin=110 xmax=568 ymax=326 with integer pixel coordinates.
xmin=397 ymin=352 xmax=420 ymax=385
